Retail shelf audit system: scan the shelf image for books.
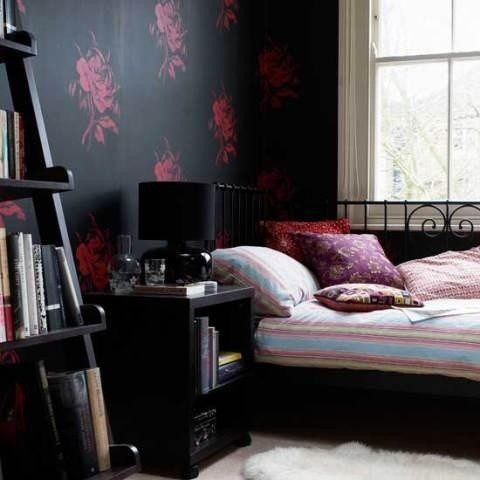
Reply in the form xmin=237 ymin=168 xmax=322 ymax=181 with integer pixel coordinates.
xmin=194 ymin=317 xmax=242 ymax=394
xmin=0 ymin=0 xmax=17 ymax=39
xmin=134 ymin=285 xmax=205 ymax=296
xmin=1 ymin=110 xmax=27 ymax=180
xmin=1 ymin=358 xmax=111 ymax=480
xmin=1 ymin=225 xmax=84 ymax=343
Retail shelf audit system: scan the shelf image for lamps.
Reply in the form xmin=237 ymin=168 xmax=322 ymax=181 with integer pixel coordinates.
xmin=137 ymin=180 xmax=217 ymax=281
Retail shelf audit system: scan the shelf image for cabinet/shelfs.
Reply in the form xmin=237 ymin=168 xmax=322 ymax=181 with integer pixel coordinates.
xmin=1 ymin=4 xmax=142 ymax=479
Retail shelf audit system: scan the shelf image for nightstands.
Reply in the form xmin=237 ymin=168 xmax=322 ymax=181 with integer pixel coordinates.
xmin=86 ymin=278 xmax=258 ymax=480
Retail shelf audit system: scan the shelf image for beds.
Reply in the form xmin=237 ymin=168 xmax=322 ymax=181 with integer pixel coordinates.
xmin=216 ymin=179 xmax=480 ymax=428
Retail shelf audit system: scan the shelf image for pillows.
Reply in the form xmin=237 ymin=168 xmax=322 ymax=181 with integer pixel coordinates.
xmin=208 ymin=217 xmax=480 ymax=319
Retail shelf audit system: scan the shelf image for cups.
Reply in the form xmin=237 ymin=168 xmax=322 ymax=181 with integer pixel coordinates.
xmin=145 ymin=257 xmax=165 ymax=287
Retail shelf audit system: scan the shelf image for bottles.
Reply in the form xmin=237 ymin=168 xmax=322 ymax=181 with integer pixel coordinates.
xmin=109 ymin=253 xmax=141 ymax=292
